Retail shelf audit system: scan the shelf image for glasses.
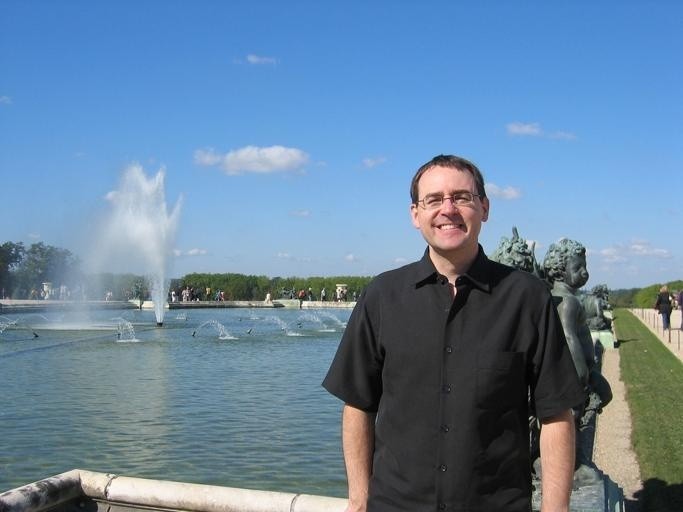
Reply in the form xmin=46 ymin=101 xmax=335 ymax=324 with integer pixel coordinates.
xmin=415 ymin=192 xmax=479 ymax=211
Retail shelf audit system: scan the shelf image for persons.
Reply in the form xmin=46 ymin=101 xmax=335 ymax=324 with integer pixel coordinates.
xmin=602 ymin=284 xmax=611 ymax=310
xmin=526 ymin=239 xmax=613 ymax=469
xmin=653 ymin=286 xmax=675 ymax=330
xmin=281 ymin=287 xmax=358 ymax=302
xmin=322 ymin=154 xmax=585 ymax=512
xmin=1 ymin=283 xmax=149 ymax=301
xmin=676 ymin=289 xmax=683 ymax=330
xmin=170 ymin=286 xmax=224 ymax=302
xmin=584 ymin=284 xmax=605 ymax=321
